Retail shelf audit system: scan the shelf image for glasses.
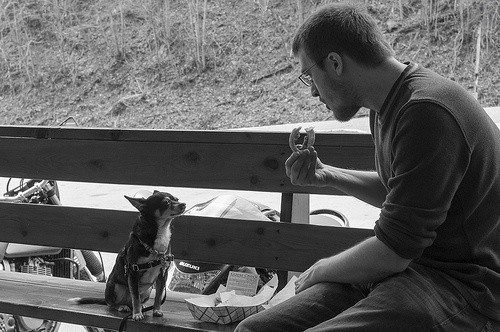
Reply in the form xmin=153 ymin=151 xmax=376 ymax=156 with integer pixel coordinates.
xmin=298 ymin=52 xmax=330 ymax=87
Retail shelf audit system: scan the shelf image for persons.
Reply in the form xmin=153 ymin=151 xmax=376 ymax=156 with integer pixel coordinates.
xmin=233 ymin=2 xmax=500 ymax=332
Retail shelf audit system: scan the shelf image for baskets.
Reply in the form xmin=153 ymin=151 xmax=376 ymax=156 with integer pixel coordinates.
xmin=3 ymin=180 xmax=60 ymax=203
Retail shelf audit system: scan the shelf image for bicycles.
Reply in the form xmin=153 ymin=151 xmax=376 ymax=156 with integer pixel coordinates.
xmin=1 ymin=114 xmax=107 ymax=332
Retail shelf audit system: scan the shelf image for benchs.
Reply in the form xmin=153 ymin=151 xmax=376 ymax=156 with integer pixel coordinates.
xmin=0 ymin=124 xmax=378 ymax=332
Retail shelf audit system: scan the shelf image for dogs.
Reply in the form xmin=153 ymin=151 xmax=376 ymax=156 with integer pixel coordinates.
xmin=68 ymin=188 xmax=187 ymax=322
